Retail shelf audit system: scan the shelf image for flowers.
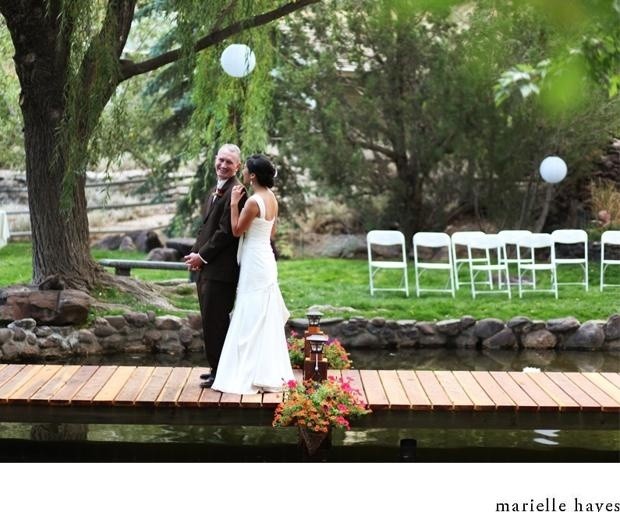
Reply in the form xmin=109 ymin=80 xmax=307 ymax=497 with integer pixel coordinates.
xmin=286 ymin=329 xmax=354 ymax=370
xmin=271 ymin=376 xmax=372 ymax=434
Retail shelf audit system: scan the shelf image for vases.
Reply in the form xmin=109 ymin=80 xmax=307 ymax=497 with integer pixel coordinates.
xmin=299 ymin=424 xmax=325 ymax=453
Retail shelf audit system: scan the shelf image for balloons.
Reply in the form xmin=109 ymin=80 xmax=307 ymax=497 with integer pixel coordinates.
xmin=219 ymin=42 xmax=257 ymax=79
xmin=537 ymin=155 xmax=569 ymax=184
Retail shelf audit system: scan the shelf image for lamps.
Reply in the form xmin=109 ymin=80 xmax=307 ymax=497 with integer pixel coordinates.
xmin=306 ymin=332 xmax=327 ymax=361
xmin=306 ymin=307 xmax=324 ymax=332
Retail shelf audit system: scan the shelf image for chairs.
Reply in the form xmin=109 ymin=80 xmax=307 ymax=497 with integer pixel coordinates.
xmin=366 ymin=228 xmax=620 ymax=300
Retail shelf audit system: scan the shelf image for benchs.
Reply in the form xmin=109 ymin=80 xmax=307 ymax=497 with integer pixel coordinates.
xmin=97 ymin=256 xmax=198 ymax=282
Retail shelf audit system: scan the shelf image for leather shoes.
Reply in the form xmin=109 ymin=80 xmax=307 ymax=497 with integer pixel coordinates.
xmin=200 ymin=374 xmax=214 ymax=388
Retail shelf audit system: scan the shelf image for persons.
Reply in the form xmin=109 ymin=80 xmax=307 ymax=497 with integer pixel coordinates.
xmin=210 ymin=154 xmax=296 ymax=397
xmin=183 ymin=142 xmax=252 ymax=388
